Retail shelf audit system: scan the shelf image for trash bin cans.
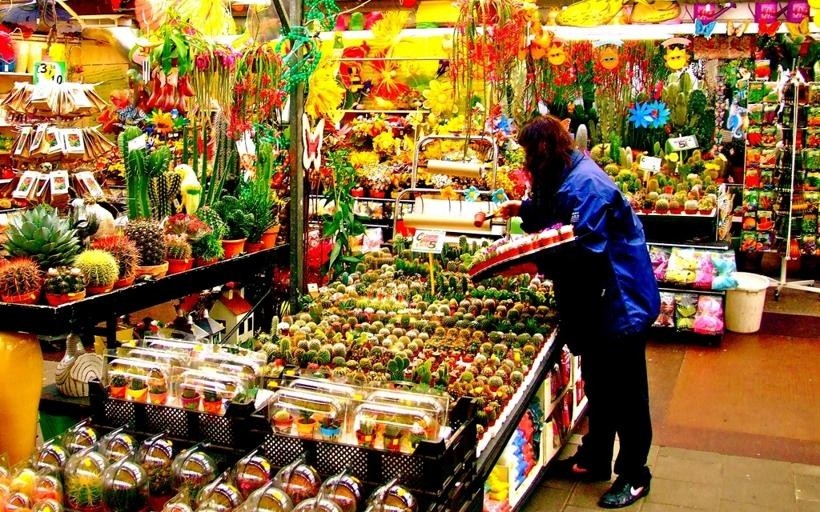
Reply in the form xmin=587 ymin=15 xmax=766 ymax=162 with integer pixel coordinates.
xmin=725 ymin=271 xmax=770 ymax=334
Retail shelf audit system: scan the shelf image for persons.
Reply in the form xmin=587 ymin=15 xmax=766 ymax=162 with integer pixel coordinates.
xmin=501 ymin=117 xmax=661 ymax=509
xmin=724 ymin=84 xmax=732 ymax=105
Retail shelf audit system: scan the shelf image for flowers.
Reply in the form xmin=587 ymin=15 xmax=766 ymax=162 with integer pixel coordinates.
xmin=166 ymin=213 xmax=210 ymax=238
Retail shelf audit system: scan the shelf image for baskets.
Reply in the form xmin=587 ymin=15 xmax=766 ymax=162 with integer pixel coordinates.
xmin=89 ymin=376 xmax=295 ymax=449
xmin=250 ymin=396 xmax=477 ymax=492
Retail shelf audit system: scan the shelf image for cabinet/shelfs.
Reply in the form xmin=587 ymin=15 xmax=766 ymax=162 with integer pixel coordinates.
xmin=648 ymin=242 xmax=734 ymax=348
xmin=311 ymin=54 xmax=449 ymax=114
xmin=1 ymin=70 xmax=37 ymax=187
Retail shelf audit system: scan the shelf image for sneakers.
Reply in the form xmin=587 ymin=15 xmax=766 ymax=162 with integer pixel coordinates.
xmin=599 ymin=477 xmax=650 ymax=508
xmin=545 ymin=456 xmax=611 ymax=483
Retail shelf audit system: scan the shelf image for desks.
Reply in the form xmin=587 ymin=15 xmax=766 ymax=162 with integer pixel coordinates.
xmin=1 ymin=235 xmax=288 ymax=365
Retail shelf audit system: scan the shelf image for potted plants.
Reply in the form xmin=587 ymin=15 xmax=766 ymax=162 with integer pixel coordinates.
xmin=1 ymin=203 xmax=80 ymax=286
xmin=44 ymin=265 xmax=88 ymax=306
xmin=215 ymin=196 xmax=249 ymax=259
xmin=245 ymin=186 xmax=270 ymax=253
xmin=620 ymin=164 xmax=715 ymax=217
xmin=193 ymin=205 xmax=224 ymax=265
xmin=165 ymin=235 xmax=193 ymax=275
xmin=109 ymin=240 xmax=138 ymax=286
xmin=124 ymin=218 xmax=169 ymax=280
xmin=0 ymin=257 xmax=42 ymax=304
xmin=75 ymin=248 xmax=119 ymax=294
xmin=262 ymin=196 xmax=281 ymax=250
